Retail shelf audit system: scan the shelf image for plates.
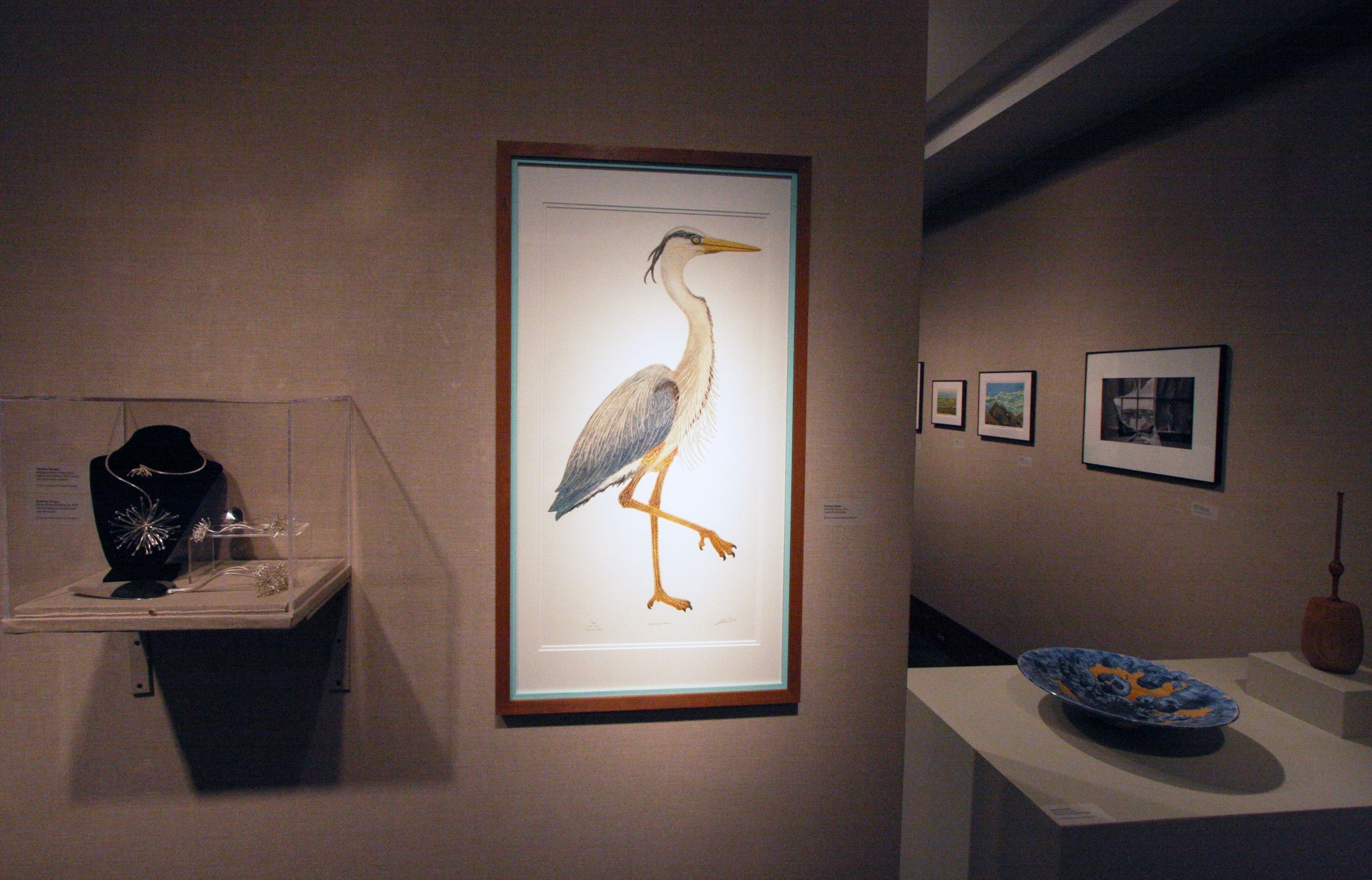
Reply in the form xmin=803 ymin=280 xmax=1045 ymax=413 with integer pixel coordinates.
xmin=1017 ymin=646 xmax=1240 ymax=730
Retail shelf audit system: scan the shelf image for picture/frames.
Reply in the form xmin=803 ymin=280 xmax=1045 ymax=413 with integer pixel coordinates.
xmin=495 ymin=141 xmax=801 ymax=711
xmin=976 ymin=370 xmax=1036 ymax=445
xmin=1082 ymin=344 xmax=1227 ymax=488
xmin=931 ymin=380 xmax=967 ymax=428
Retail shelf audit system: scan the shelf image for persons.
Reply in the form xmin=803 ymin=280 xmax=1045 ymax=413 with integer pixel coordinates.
xmin=89 ymin=425 xmax=222 ymax=585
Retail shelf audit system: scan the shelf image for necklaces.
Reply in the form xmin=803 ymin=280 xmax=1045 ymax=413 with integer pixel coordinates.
xmin=104 ymin=444 xmax=208 ymax=557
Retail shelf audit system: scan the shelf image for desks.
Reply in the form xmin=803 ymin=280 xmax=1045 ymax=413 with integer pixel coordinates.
xmin=905 ymin=647 xmax=1371 ymax=879
